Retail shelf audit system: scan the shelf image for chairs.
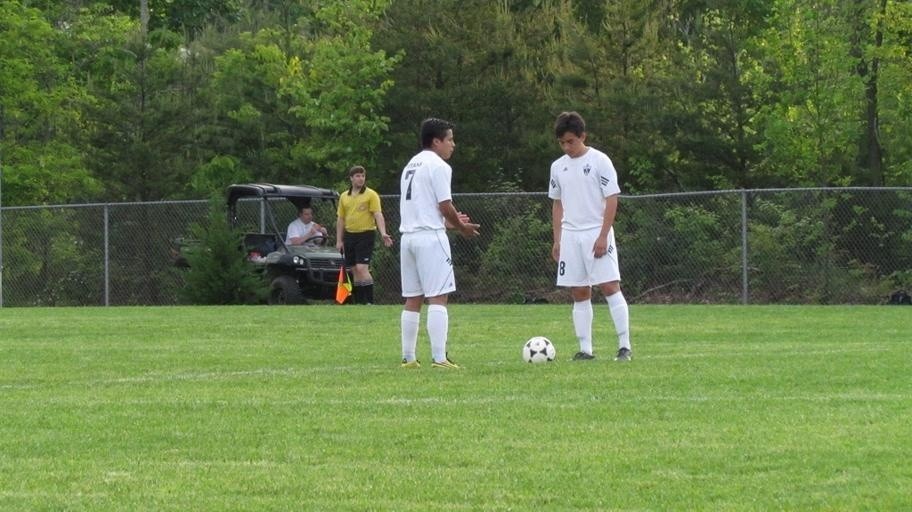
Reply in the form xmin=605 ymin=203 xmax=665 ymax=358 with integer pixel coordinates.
xmin=243 ymin=232 xmax=287 ymax=271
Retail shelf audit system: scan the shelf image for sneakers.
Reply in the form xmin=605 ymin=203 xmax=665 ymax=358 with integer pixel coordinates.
xmin=615 ymin=348 xmax=632 ymax=361
xmin=401 ymin=352 xmax=465 ymax=369
xmin=569 ymin=352 xmax=596 ymax=361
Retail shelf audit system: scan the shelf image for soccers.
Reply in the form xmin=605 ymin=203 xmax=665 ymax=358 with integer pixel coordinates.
xmin=523 ymin=336 xmax=556 ymax=363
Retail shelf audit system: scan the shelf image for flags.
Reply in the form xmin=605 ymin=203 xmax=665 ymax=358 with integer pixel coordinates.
xmin=335 ymin=264 xmax=353 ymax=304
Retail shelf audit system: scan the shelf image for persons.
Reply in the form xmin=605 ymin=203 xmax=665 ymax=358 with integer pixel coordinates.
xmin=285 ymin=206 xmax=328 ymax=245
xmin=548 ymin=111 xmax=633 ymax=361
xmin=398 ymin=118 xmax=481 ymax=369
xmin=336 ymin=166 xmax=394 ymax=305
